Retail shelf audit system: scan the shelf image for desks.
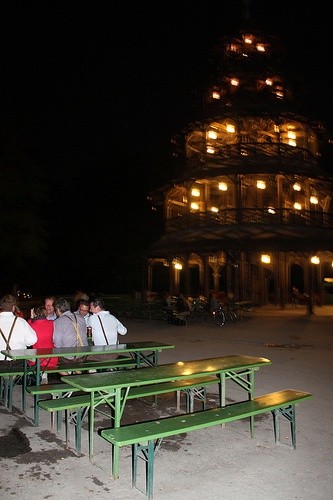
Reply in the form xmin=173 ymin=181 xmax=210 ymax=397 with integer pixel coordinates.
xmin=61 ymin=354 xmax=272 ymax=480
xmin=1 ymin=339 xmax=175 ymax=426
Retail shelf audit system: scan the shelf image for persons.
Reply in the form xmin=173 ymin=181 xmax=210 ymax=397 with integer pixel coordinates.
xmin=292 ymin=287 xmax=313 ymax=316
xmin=0 ymin=288 xmax=127 ymax=403
xmin=173 ymin=288 xmax=236 ymax=321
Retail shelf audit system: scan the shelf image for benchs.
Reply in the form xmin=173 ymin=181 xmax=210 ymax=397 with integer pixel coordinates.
xmin=1 ymin=358 xmax=314 ymax=499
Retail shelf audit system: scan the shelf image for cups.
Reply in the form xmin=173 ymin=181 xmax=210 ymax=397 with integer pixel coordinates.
xmin=87 ymin=327 xmax=92 ymax=337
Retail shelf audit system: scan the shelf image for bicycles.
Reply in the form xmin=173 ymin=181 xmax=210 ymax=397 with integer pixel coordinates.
xmin=188 ymin=295 xmax=239 ymax=328
